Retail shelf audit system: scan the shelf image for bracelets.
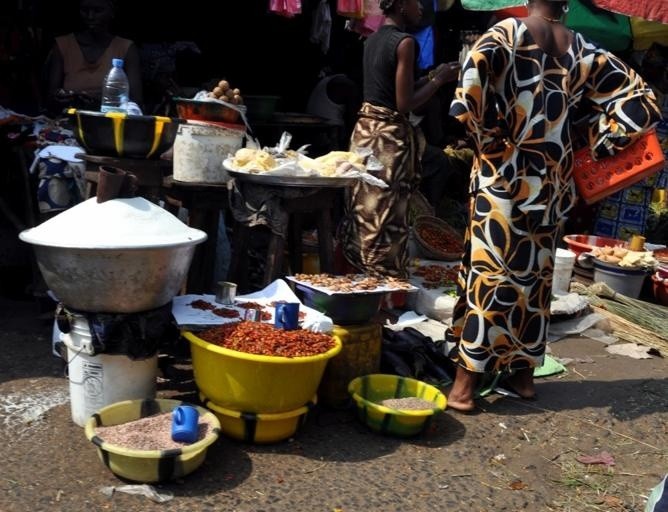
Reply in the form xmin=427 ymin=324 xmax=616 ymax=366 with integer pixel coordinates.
xmin=427 ymin=70 xmax=438 ymax=80
xmin=432 ymin=76 xmax=442 ymax=88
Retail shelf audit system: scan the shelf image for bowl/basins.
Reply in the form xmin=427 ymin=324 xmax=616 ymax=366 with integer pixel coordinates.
xmin=177 ymin=322 xmax=342 ymax=413
xmin=85 ymin=403 xmax=221 ymax=480
xmin=348 ymin=375 xmax=445 ymax=434
xmin=296 ymin=284 xmax=382 ymax=327
xmin=562 ymin=233 xmax=629 ymax=261
xmin=20 ymin=198 xmax=210 ymax=312
xmin=200 ymin=397 xmax=318 ymax=442
xmin=60 ymin=99 xmax=244 ymax=158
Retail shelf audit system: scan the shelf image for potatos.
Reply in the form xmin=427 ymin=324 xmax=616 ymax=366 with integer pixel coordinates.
xmin=210 ymin=80 xmax=242 ymax=105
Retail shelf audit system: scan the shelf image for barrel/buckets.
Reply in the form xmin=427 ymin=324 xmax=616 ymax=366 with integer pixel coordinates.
xmin=59 ymin=314 xmax=160 ymax=429
xmin=552 ymin=248 xmax=576 ymax=295
xmin=589 ymin=258 xmax=650 ymax=298
xmin=171 ymin=120 xmax=247 ymax=183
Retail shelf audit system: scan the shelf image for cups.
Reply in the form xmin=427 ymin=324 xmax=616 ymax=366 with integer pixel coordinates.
xmin=215 ymin=282 xmax=237 ymax=305
xmin=171 ymin=405 xmax=199 ymax=442
xmin=95 ymin=160 xmax=139 ymax=203
xmin=275 ymin=302 xmax=300 ymax=331
xmin=631 ymin=234 xmax=646 ymax=251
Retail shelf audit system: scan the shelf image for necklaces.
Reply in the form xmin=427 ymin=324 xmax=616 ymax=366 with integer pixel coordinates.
xmin=531 ymin=15 xmax=560 ymax=22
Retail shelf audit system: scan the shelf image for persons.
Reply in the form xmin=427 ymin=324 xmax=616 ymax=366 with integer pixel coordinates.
xmin=439 ymin=0 xmax=663 ymax=414
xmin=338 ymin=0 xmax=462 ymax=274
xmin=37 ymin=0 xmax=142 ymax=111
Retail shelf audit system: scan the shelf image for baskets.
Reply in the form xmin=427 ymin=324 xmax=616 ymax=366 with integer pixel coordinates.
xmin=570 ymin=126 xmax=665 ymax=205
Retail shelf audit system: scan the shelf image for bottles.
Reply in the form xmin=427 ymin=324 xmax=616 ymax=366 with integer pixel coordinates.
xmin=102 ymin=57 xmax=129 ymax=114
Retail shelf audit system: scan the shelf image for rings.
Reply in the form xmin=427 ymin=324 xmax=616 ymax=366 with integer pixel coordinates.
xmin=451 ymin=66 xmax=457 ymax=73
